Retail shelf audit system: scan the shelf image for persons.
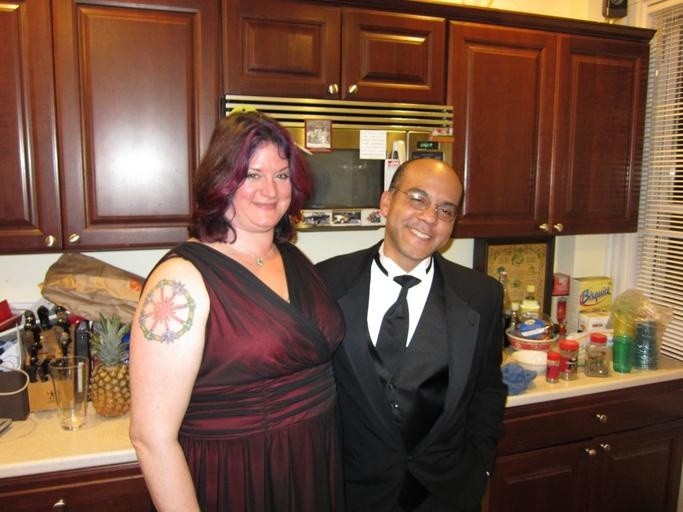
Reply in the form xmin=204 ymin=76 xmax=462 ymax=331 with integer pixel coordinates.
xmin=314 ymin=157 xmax=509 ymax=511
xmin=129 ymin=112 xmax=346 ymax=511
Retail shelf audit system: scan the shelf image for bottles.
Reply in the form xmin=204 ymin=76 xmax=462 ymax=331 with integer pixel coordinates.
xmin=496 ymin=269 xmax=659 ymax=383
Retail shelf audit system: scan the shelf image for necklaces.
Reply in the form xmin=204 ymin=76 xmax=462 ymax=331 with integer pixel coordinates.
xmin=233 ymin=244 xmax=275 ymax=266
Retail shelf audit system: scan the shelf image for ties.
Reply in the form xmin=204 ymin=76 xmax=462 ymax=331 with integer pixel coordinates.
xmin=376 ymin=275 xmax=421 ymax=365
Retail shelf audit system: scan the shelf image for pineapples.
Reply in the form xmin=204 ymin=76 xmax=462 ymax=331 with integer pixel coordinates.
xmin=79 ymin=312 xmax=132 ymax=419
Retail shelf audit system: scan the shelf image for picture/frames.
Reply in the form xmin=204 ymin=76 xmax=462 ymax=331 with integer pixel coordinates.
xmin=472 ymin=237 xmax=555 ymax=327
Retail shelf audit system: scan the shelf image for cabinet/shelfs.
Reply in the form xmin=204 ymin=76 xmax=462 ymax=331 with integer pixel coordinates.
xmin=220 ymin=0 xmax=449 ymax=104
xmin=487 ymin=376 xmax=683 ymax=512
xmin=0 ymin=459 xmax=157 ymax=512
xmin=0 ymin=0 xmax=221 ymax=255
xmin=449 ymin=3 xmax=657 ymax=238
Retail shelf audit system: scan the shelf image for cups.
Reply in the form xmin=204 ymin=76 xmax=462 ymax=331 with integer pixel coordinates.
xmin=47 ymin=356 xmax=89 ymax=432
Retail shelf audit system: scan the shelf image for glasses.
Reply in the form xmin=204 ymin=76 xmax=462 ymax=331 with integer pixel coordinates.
xmin=392 ymin=187 xmax=457 ymax=222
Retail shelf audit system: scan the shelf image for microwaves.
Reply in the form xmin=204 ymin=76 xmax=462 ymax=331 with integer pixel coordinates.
xmin=220 ymin=96 xmax=455 ymax=232
